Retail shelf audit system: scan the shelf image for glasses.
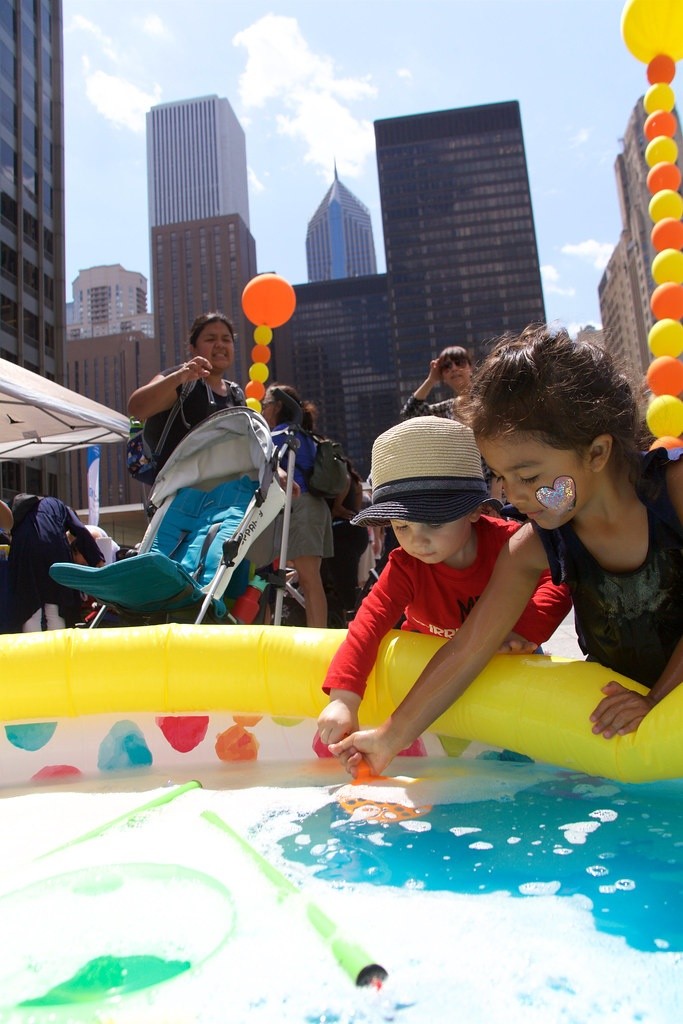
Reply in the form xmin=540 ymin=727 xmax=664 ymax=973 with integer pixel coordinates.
xmin=440 ymin=358 xmax=468 ymax=368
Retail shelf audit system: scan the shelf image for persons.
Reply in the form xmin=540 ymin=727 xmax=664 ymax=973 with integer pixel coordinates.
xmin=71 ymin=538 xmax=104 ymax=622
xmin=261 ymin=381 xmax=334 ymax=629
xmin=59 ymin=505 xmax=121 ymax=565
xmin=0 ymin=500 xmax=13 ymax=535
xmin=326 ymin=323 xmax=683 ymax=778
xmin=316 ymin=415 xmax=575 ymax=747
xmin=6 ymin=493 xmax=103 ymax=634
xmin=116 ymin=548 xmax=138 ymax=562
xmin=400 ymin=346 xmax=512 ymax=508
xmin=351 ymin=482 xmax=386 ymax=589
xmin=317 ymin=439 xmax=368 ymax=626
xmin=129 ymin=313 xmax=300 ymax=506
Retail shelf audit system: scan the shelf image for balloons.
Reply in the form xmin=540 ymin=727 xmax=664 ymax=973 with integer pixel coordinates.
xmin=241 ymin=273 xmax=297 ymax=328
xmin=651 ymin=248 xmax=683 ymax=286
xmin=643 ymin=109 xmax=677 ymax=142
xmin=251 ymin=345 xmax=271 ymax=364
xmin=248 ymin=363 xmax=269 ymax=383
xmin=649 ymin=436 xmax=683 ymax=453
xmin=620 ymin=0 xmax=683 ymax=63
xmin=650 ymin=281 xmax=682 ymax=321
xmin=254 ymin=325 xmax=273 ymax=345
xmin=648 ymin=189 xmax=683 ymax=224
xmin=645 ymin=135 xmax=679 ymax=170
xmin=645 ymin=54 xmax=677 ymax=85
xmin=647 ymin=394 xmax=683 ymax=439
xmin=244 ymin=381 xmax=265 ymax=401
xmin=646 ymin=161 xmax=681 ymax=195
xmin=647 ymin=355 xmax=683 ymax=397
xmin=643 ymin=81 xmax=675 ymax=113
xmin=244 ymin=398 xmax=262 ymax=414
xmin=650 ymin=217 xmax=683 ymax=252
xmin=648 ymin=318 xmax=683 ymax=358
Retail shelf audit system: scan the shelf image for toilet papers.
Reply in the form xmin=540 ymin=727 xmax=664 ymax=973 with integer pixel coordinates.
xmin=95 ymin=536 xmax=121 ymax=566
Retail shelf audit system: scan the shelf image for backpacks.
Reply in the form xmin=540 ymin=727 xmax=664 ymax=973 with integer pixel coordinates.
xmin=127 ymin=370 xmax=245 ymax=485
xmin=287 ymin=425 xmax=347 ymax=498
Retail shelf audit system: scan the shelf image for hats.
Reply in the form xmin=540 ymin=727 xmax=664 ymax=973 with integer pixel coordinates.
xmin=346 ymin=417 xmax=486 ymax=527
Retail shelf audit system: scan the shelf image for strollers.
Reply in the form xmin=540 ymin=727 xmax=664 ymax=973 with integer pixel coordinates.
xmin=48 ymin=405 xmax=308 ymax=626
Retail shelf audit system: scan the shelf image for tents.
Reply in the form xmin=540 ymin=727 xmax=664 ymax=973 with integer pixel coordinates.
xmin=1 ymin=357 xmax=133 ymax=463
